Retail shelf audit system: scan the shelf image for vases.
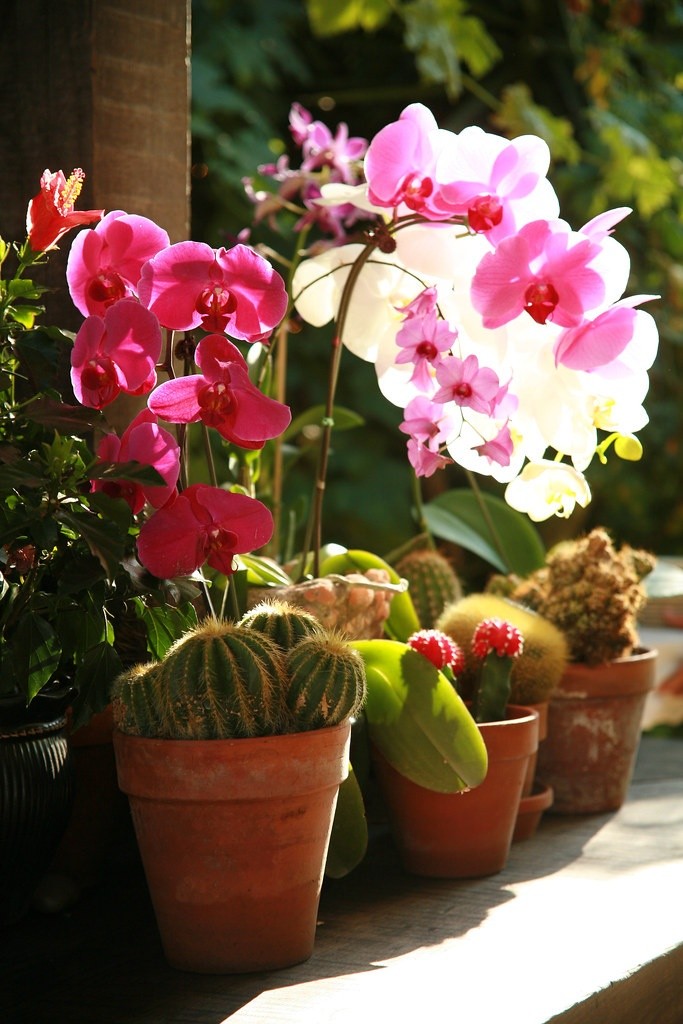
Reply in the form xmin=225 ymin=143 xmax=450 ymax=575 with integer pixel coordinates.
xmin=1 ymin=700 xmax=70 ymax=928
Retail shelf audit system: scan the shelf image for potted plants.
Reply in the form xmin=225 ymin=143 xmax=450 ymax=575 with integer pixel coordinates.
xmin=386 ymin=594 xmax=565 ymax=882
xmin=486 ymin=521 xmax=657 ymax=817
xmin=112 ymin=623 xmax=371 ymax=980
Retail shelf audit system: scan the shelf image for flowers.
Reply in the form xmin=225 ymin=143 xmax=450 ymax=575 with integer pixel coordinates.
xmin=0 ymin=103 xmax=665 ymax=733
